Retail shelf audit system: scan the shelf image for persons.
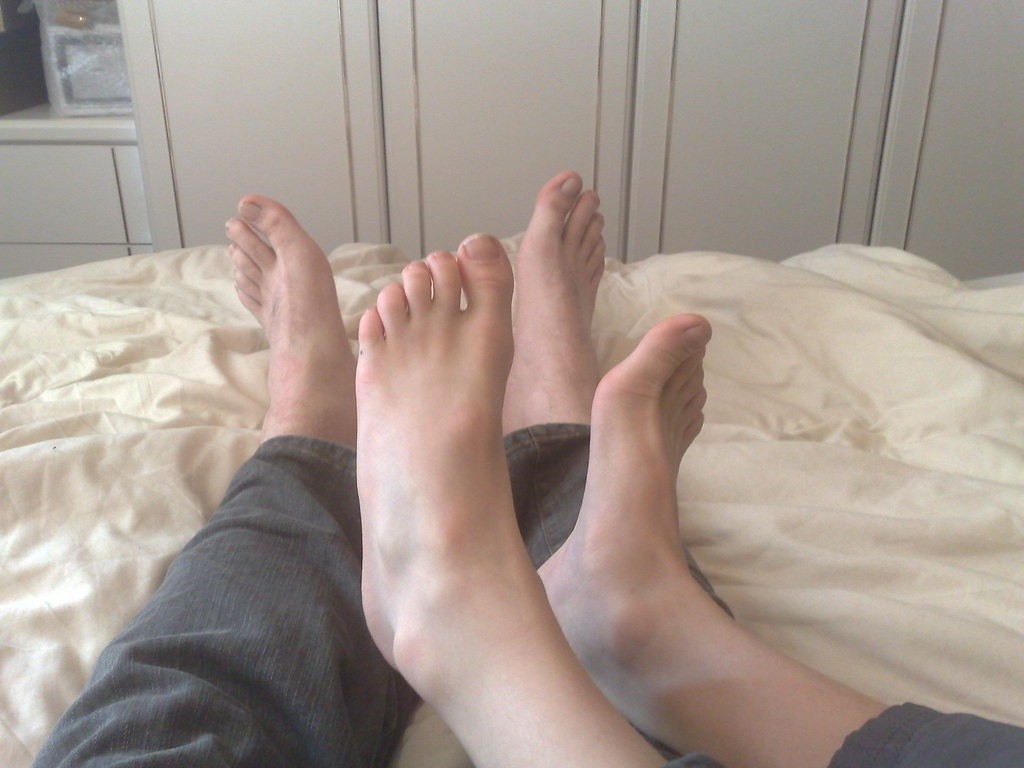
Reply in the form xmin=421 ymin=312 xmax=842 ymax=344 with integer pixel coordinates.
xmin=354 ymin=234 xmax=1024 ymax=768
xmin=32 ymin=171 xmax=726 ymax=768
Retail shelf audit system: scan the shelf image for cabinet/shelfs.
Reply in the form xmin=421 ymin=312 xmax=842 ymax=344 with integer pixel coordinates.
xmin=116 ymin=0 xmax=641 ymax=264
xmin=1 ymin=101 xmax=155 ymax=277
xmin=628 ymin=1 xmax=1024 ymax=283
